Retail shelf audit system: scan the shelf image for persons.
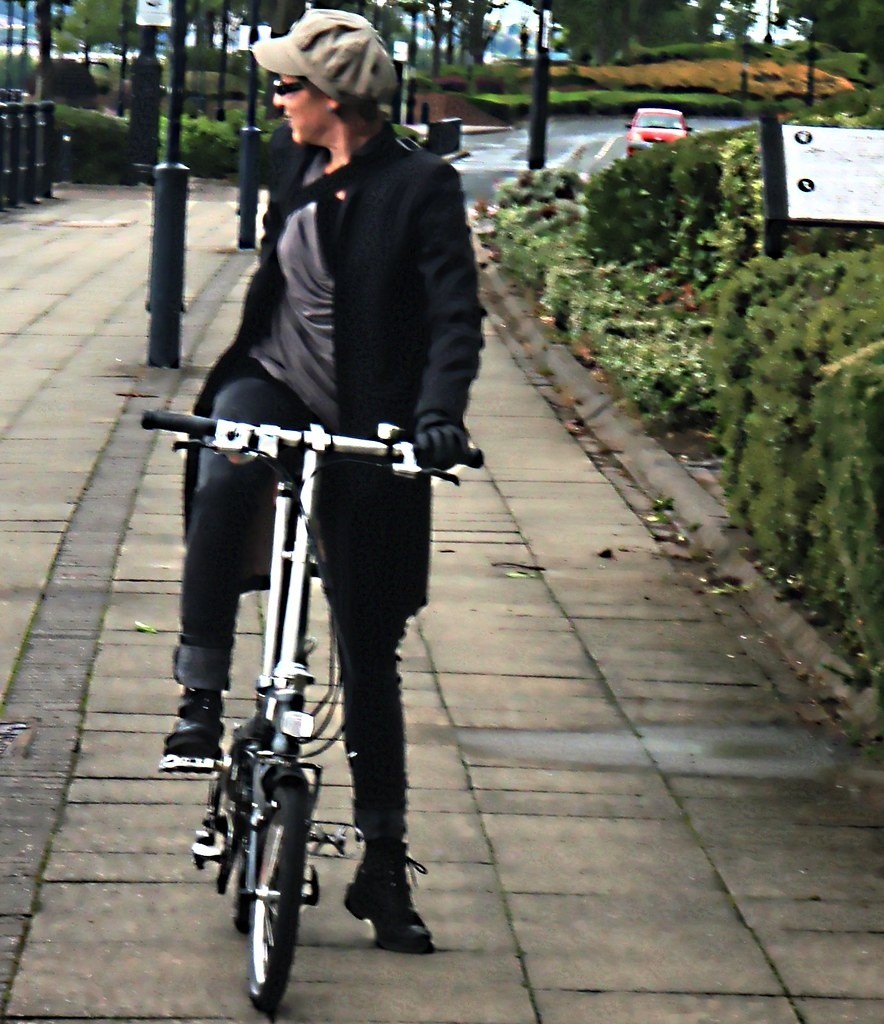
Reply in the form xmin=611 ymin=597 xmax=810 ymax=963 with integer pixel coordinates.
xmin=158 ymin=9 xmax=485 ymax=954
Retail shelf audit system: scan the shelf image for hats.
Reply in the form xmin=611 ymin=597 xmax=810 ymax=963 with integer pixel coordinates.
xmin=252 ymin=9 xmax=398 ymax=107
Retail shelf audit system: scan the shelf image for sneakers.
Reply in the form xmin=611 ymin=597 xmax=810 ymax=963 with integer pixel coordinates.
xmin=345 ymin=840 xmax=431 ymax=953
xmin=162 ymin=689 xmax=224 ymax=774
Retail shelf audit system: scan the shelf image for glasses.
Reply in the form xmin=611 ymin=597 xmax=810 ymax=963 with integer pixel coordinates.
xmin=273 ymin=78 xmax=304 ymax=96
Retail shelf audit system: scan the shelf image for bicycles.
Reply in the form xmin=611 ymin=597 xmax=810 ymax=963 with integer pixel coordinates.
xmin=132 ymin=405 xmax=486 ymax=1010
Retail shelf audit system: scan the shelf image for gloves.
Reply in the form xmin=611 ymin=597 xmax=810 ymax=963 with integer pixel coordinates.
xmin=415 ymin=423 xmax=469 ymax=471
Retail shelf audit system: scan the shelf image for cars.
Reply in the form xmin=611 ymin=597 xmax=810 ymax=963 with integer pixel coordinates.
xmin=623 ymin=108 xmax=693 ymax=159
